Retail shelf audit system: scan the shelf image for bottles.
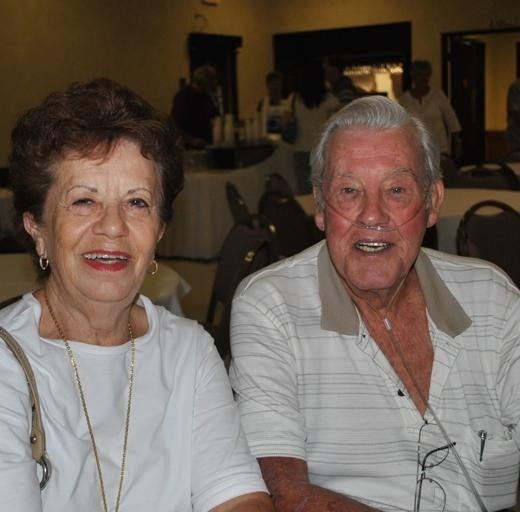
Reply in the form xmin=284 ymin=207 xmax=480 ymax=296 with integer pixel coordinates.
xmin=211 ymin=108 xmax=266 ymax=148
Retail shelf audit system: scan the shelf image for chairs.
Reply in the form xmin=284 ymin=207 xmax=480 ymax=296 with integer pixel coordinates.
xmin=444 ymin=160 xmax=519 ymax=191
xmin=206 ymin=174 xmax=310 ymax=346
xmin=420 ymin=220 xmax=439 ymax=253
xmin=457 ymin=202 xmax=519 ymax=289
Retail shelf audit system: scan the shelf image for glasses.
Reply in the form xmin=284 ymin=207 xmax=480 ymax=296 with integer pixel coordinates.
xmin=414 ymin=423 xmax=455 ymax=512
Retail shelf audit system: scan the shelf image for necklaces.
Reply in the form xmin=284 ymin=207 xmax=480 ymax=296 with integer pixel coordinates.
xmin=45 ymin=288 xmax=135 ymax=511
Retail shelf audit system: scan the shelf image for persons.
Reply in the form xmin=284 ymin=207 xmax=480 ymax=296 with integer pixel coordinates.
xmin=256 ymin=51 xmax=361 ymax=167
xmin=228 ymin=98 xmax=520 ymax=511
xmin=507 ymin=68 xmax=520 ymax=143
xmin=169 ymin=66 xmax=219 ymax=152
xmin=397 ymin=60 xmax=462 ymax=164
xmin=2 ymin=80 xmax=274 ymax=512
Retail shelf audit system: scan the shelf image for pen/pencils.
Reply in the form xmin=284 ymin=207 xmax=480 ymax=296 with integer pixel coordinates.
xmin=478 ymin=430 xmax=487 ymax=462
